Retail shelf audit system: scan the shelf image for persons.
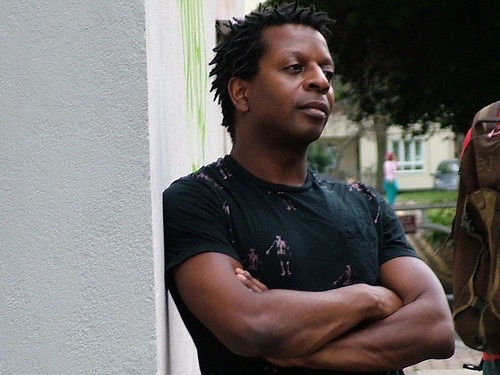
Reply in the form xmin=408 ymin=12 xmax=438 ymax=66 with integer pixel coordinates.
xmin=162 ymin=0 xmax=456 ymax=375
xmin=383 ymin=152 xmax=399 ymax=208
xmin=453 ymin=101 xmax=500 ymax=375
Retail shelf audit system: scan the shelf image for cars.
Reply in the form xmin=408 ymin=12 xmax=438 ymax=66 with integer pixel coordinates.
xmin=431 ymin=159 xmax=461 ymax=191
